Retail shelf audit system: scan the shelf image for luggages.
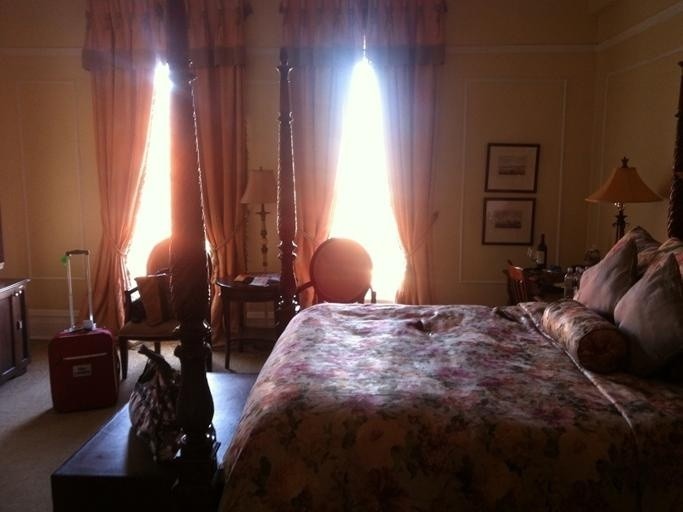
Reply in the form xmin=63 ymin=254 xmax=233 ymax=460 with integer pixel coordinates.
xmin=47 ymin=249 xmax=120 ymax=414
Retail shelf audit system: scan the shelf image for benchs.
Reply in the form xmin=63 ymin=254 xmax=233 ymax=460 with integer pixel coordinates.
xmin=52 ymin=371 xmax=258 ymax=512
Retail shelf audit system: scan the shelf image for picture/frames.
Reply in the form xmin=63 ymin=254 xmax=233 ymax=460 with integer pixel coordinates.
xmin=482 ymin=198 xmax=535 ymax=245
xmin=485 ymin=143 xmax=539 ymax=193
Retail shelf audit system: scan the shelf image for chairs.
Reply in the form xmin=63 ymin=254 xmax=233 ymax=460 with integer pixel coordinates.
xmin=507 ymin=265 xmax=529 ymax=306
xmin=293 ymin=238 xmax=376 ymax=313
xmin=117 ymin=237 xmax=215 ymax=379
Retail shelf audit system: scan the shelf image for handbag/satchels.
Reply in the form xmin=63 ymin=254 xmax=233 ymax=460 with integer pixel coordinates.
xmin=128 ymin=344 xmax=221 ymax=497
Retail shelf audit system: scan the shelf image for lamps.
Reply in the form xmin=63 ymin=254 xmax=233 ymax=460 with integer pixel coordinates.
xmin=585 ymin=157 xmax=663 ymax=243
xmin=240 ymin=166 xmax=277 ymax=319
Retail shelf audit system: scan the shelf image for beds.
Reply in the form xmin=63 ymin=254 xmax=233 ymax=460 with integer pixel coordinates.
xmin=219 ymin=302 xmax=683 ymax=512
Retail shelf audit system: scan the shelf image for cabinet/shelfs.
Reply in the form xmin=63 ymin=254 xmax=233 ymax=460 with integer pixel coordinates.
xmin=0 ymin=278 xmax=32 ymax=383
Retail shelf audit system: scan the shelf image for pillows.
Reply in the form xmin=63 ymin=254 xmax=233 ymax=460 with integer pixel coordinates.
xmin=134 ymin=273 xmax=173 ymax=327
xmin=542 ymin=227 xmax=683 ymax=377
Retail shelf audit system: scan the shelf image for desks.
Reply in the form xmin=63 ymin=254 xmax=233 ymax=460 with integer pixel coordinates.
xmin=216 ymin=272 xmax=298 ymax=369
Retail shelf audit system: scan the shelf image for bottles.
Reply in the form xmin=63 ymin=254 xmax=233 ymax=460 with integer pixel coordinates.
xmin=535 ymin=233 xmax=548 ymax=270
xmin=563 ymin=266 xmax=591 ymax=299
